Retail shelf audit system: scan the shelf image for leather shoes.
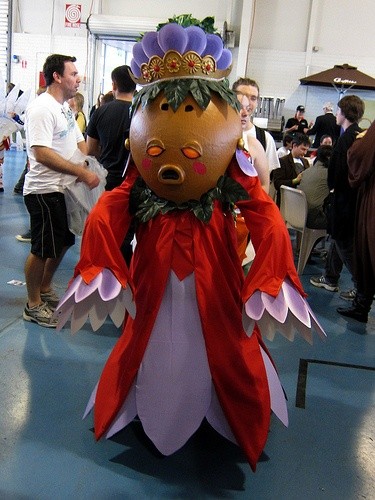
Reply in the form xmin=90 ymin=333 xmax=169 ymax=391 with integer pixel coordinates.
xmin=336 ymin=306 xmax=369 ymax=323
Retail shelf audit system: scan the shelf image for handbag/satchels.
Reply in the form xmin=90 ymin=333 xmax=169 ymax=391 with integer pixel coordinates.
xmin=306 ymin=206 xmax=327 ymax=229
xmin=64 ymin=156 xmax=109 ymax=236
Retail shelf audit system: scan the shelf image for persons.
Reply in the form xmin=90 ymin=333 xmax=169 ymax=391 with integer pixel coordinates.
xmin=23 ymin=51 xmax=102 ymax=329
xmin=84 ymin=63 xmax=142 ymax=268
xmin=310 ymin=95 xmax=366 ymax=299
xmin=231 ymin=78 xmax=339 ymax=265
xmin=336 ymin=122 xmax=375 ymax=322
xmin=0 ymin=84 xmax=114 ymax=243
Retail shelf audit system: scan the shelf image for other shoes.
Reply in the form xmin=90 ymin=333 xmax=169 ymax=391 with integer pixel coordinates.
xmin=16 ymin=230 xmax=31 ymax=241
xmin=14 ymin=188 xmax=23 ymax=194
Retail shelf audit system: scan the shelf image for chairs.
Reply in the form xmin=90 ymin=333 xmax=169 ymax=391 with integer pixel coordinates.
xmin=280 ymin=185 xmax=329 ymax=276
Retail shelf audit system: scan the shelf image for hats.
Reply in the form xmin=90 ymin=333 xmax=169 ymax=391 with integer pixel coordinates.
xmin=297 ymin=105 xmax=305 ymax=111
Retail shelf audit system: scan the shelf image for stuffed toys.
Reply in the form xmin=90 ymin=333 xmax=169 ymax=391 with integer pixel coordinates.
xmin=57 ymin=22 xmax=326 ymax=463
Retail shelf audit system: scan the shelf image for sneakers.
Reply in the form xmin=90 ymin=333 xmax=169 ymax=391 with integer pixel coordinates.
xmin=23 ymin=303 xmax=58 ymax=327
xmin=309 ymin=275 xmax=340 ymax=292
xmin=41 ymin=292 xmax=61 ymax=311
xmin=340 ymin=288 xmax=358 ymax=301
